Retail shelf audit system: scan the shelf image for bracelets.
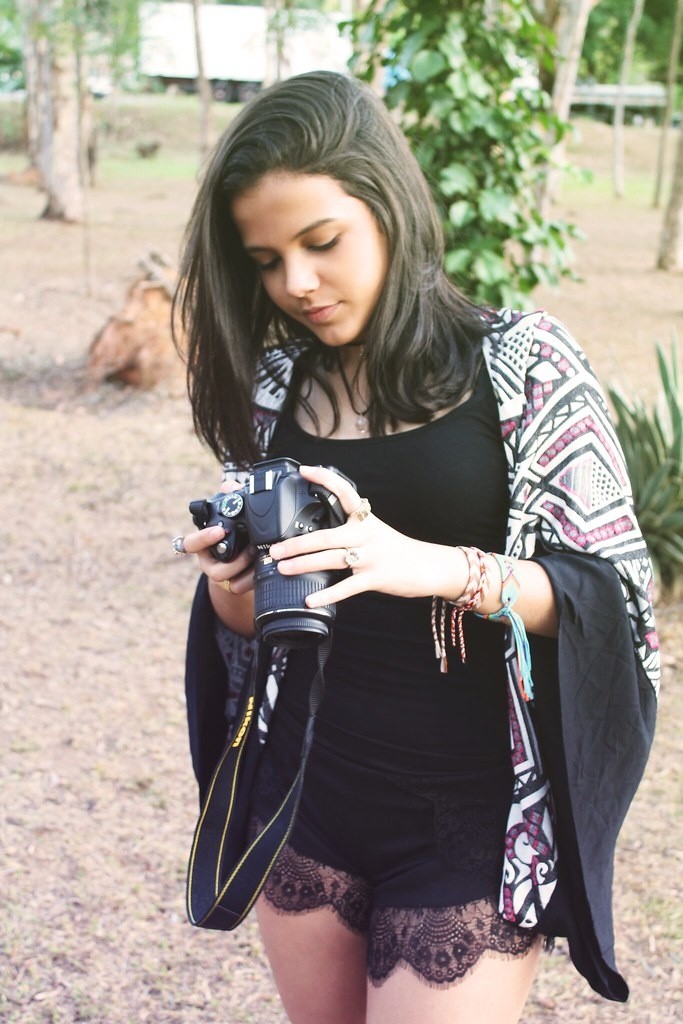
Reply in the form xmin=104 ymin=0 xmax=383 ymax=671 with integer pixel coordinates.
xmin=429 ymin=543 xmax=537 ymax=702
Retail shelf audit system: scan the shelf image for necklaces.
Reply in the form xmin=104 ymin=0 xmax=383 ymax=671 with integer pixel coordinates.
xmin=353 ymin=343 xmax=375 ymax=434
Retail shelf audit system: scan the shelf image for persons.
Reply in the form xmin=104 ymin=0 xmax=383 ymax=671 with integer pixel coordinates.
xmin=169 ymin=70 xmax=677 ymax=1024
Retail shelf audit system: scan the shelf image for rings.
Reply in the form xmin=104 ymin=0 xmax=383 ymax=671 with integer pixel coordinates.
xmin=342 ymin=546 xmax=361 ymax=569
xmin=219 ymin=579 xmax=236 ymax=596
xmin=348 ymin=498 xmax=372 ymax=522
xmin=171 ymin=534 xmax=188 ymax=556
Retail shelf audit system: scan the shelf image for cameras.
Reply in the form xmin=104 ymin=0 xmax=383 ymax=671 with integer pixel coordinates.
xmin=189 ymin=456 xmax=362 ymax=652
